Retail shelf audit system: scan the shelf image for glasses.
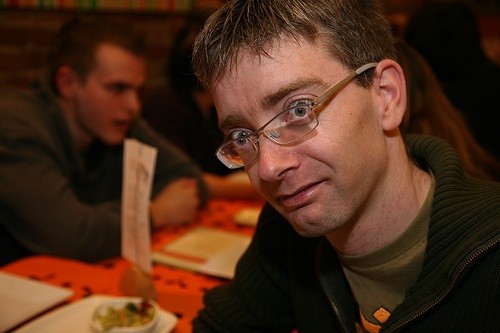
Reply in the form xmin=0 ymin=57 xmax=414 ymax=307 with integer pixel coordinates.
xmin=216 ymin=63 xmax=378 ymax=168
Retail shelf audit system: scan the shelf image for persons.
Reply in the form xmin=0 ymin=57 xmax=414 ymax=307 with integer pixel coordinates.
xmin=0 ymin=17 xmax=209 ymax=265
xmin=150 ymin=0 xmax=500 ymax=187
xmin=192 ymin=0 xmax=500 ymax=333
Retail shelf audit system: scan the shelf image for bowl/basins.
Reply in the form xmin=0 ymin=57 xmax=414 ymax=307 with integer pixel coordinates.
xmin=89 ymin=298 xmax=161 ymax=333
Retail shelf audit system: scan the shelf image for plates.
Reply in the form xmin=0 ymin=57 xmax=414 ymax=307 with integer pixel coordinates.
xmin=0 ymin=273 xmax=75 ymax=333
xmin=13 ymin=295 xmax=179 ymax=333
